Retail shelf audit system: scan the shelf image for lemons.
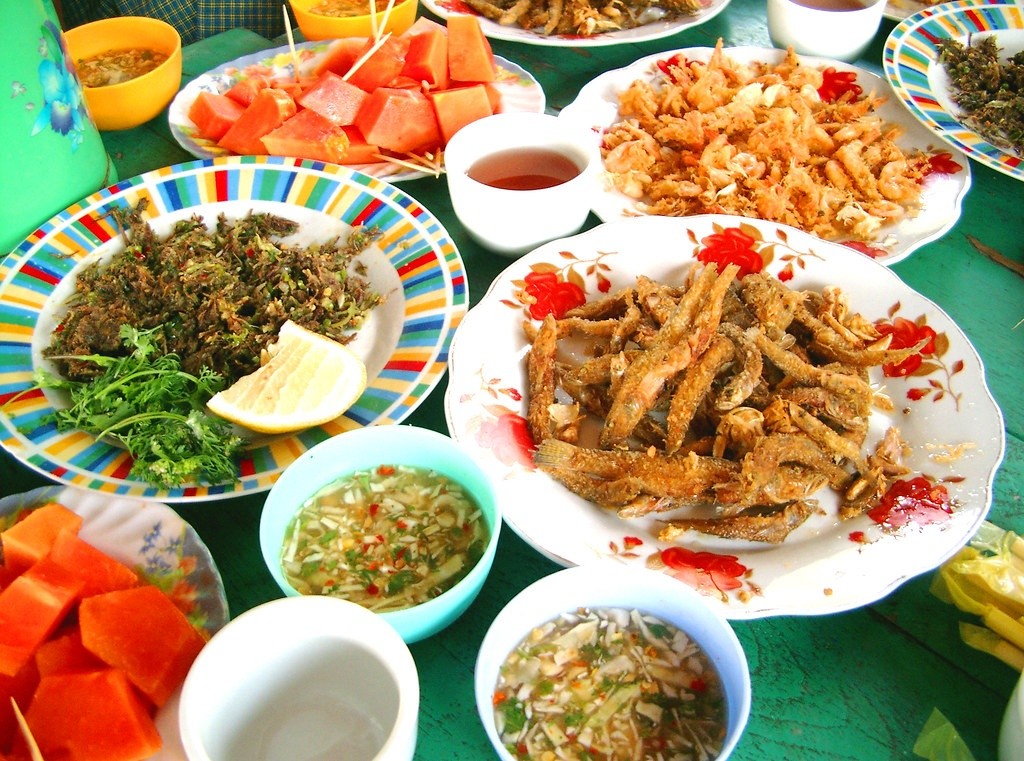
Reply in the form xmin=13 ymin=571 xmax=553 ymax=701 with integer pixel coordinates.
xmin=205 ymin=321 xmax=369 ymax=433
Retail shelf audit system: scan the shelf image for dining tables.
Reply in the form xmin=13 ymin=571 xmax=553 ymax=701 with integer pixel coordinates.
xmin=1 ymin=0 xmax=1024 ymax=760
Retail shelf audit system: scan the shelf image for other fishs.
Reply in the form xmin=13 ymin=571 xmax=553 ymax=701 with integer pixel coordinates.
xmin=528 ymin=257 xmax=911 ymax=544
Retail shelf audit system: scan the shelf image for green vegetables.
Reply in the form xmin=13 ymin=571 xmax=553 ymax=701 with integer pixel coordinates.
xmin=0 ymin=323 xmax=252 ymax=491
xmin=284 ymin=476 xmax=485 ymax=602
xmin=935 ymin=38 xmax=1024 ymax=148
xmin=498 ymin=625 xmax=726 ymax=761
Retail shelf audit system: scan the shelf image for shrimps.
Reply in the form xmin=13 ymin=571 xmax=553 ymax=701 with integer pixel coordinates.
xmin=598 ymin=35 xmax=932 ymax=242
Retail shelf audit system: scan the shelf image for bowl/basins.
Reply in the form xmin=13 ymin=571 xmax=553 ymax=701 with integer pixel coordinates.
xmin=288 ymin=0 xmax=418 ymax=41
xmin=474 ymin=564 xmax=751 ymax=761
xmin=259 ymin=424 xmax=503 ymax=644
xmin=63 ymin=16 xmax=181 ymax=130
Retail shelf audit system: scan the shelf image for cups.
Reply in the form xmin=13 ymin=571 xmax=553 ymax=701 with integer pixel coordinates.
xmin=444 ymin=112 xmax=595 ymax=257
xmin=766 ymin=0 xmax=886 ymax=64
xmin=177 ymin=596 xmax=420 ymax=761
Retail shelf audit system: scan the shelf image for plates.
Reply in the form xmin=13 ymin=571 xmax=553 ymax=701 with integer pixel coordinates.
xmin=443 ymin=214 xmax=1005 ymax=621
xmin=0 ymin=486 xmax=230 ymax=761
xmin=883 ymin=0 xmax=1024 ymax=182
xmin=0 ymin=155 xmax=470 ymax=504
xmin=169 ymin=38 xmax=546 ymax=183
xmin=880 ymin=0 xmax=904 ymax=22
xmin=420 ymin=0 xmax=732 ymax=47
xmin=557 ymin=46 xmax=972 ymax=266
